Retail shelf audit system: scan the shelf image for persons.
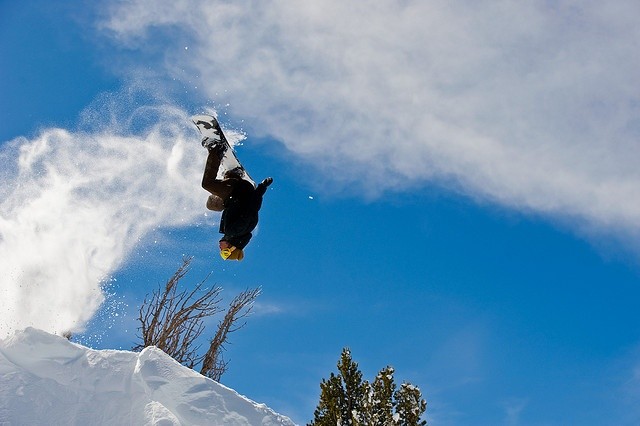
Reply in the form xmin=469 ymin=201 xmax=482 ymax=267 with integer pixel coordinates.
xmin=201 ymin=136 xmax=274 ymax=260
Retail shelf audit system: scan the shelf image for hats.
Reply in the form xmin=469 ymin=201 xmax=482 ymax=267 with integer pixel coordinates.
xmin=221 ymin=245 xmax=244 ymax=261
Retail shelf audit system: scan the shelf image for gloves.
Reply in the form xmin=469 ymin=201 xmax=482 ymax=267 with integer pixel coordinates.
xmin=259 ymin=177 xmax=273 ymax=192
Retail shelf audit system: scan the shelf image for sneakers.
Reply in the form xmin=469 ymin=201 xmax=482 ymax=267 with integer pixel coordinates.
xmin=221 ymin=167 xmax=245 ymax=180
xmin=201 ymin=137 xmax=228 ymax=153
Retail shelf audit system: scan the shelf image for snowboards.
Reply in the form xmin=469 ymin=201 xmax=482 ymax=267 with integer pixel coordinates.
xmin=192 ymin=115 xmax=256 ymax=187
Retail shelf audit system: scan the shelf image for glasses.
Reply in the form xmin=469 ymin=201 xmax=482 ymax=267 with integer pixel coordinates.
xmin=221 ymin=246 xmax=236 ymax=261
